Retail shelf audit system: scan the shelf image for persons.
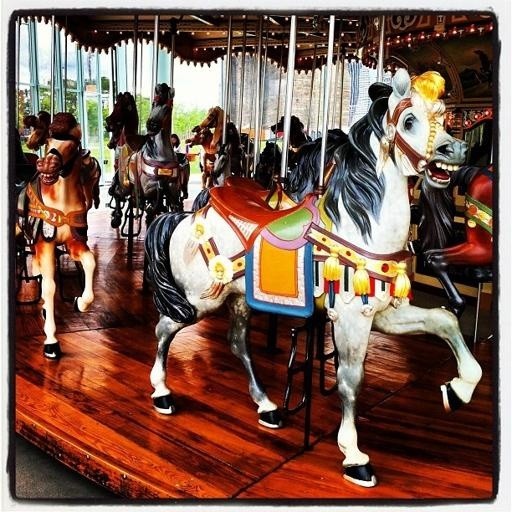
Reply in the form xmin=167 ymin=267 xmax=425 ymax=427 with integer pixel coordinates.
xmin=171 ymin=134 xmax=182 ymax=153
